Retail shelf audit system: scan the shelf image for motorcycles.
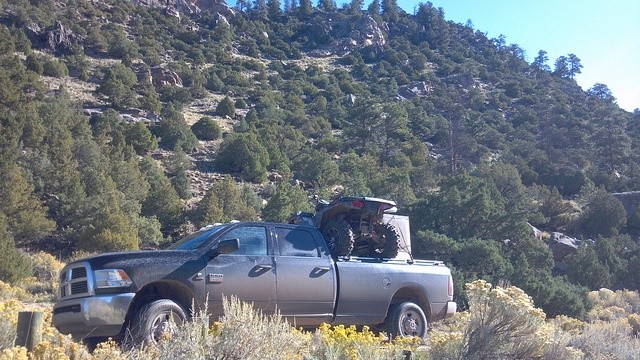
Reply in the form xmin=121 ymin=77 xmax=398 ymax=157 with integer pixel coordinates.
xmin=288 ymin=192 xmax=402 ymax=263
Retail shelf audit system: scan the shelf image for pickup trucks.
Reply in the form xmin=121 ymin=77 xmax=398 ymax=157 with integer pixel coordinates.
xmin=50 ymin=209 xmax=458 ymax=354
